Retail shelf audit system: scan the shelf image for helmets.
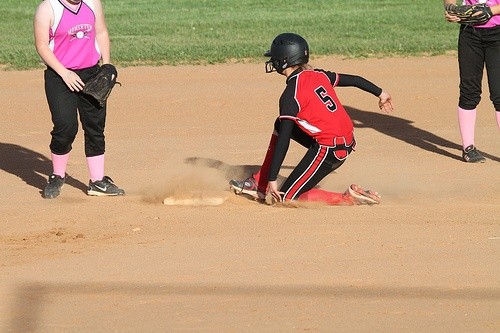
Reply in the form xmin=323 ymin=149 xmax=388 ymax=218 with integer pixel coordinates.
xmin=264 ymin=33 xmax=309 ymax=72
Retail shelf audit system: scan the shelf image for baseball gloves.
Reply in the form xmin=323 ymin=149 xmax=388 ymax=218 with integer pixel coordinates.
xmin=445 ymin=3 xmax=493 ymax=26
xmin=78 ymin=64 xmax=120 ymax=110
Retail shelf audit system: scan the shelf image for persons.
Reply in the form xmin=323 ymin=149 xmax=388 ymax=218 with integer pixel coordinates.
xmin=34 ymin=0 xmax=125 ymax=199
xmin=229 ymin=33 xmax=395 ymax=205
xmin=445 ymin=0 xmax=500 ymax=162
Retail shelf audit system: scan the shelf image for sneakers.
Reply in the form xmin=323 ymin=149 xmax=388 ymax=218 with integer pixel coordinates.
xmin=87 ymin=176 xmax=125 ymax=196
xmin=462 ymin=144 xmax=486 ymax=163
xmin=342 ymin=184 xmax=382 ymax=206
xmin=228 ymin=177 xmax=265 ymax=200
xmin=42 ymin=173 xmax=66 ymax=199
